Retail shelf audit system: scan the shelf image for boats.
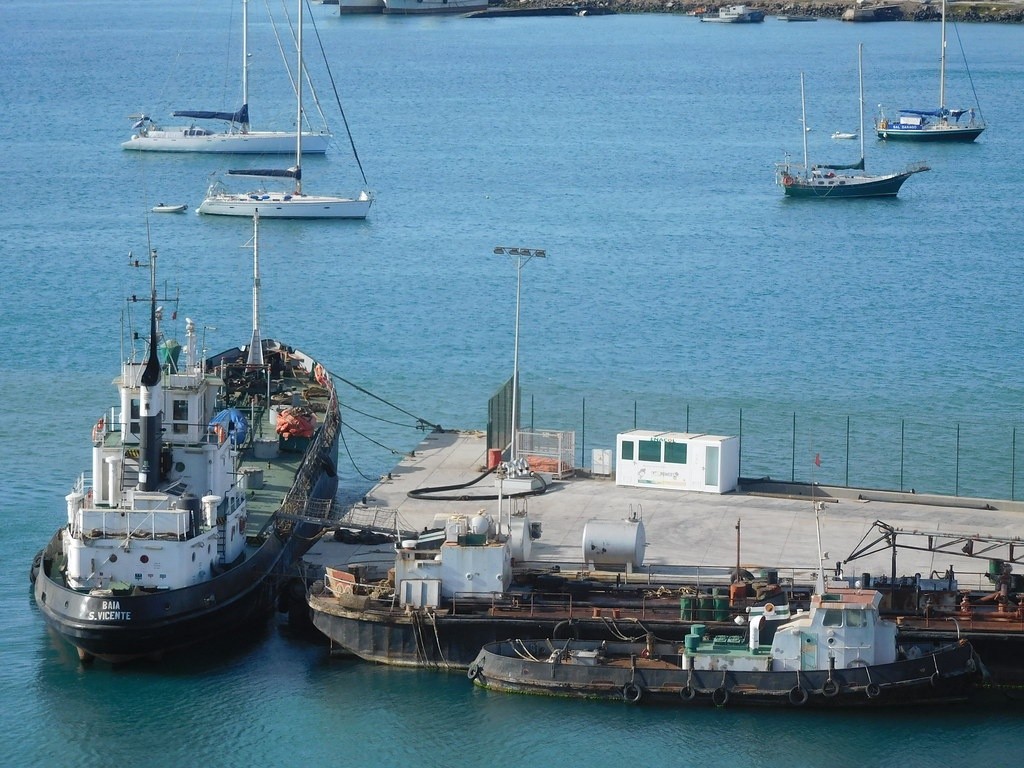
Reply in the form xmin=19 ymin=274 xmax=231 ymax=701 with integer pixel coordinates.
xmin=338 ymin=0 xmax=386 ymax=15
xmin=776 ymin=15 xmax=787 ymax=20
xmin=29 ymin=209 xmax=343 ymax=667
xmin=786 ymin=15 xmax=818 ymax=21
xmin=698 ymin=4 xmax=766 ymax=23
xmin=150 ymin=203 xmax=188 ymax=213
xmin=322 ymin=0 xmax=339 ymax=4
xmin=468 ymin=496 xmax=988 ymax=720
xmin=382 ymin=0 xmax=489 ymax=14
xmin=295 ymin=249 xmax=1024 ymax=710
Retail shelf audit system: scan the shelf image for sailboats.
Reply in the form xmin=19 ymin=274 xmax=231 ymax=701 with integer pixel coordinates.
xmin=120 ymin=0 xmax=333 ymax=154
xmin=196 ymin=0 xmax=375 ymax=220
xmin=773 ymin=43 xmax=932 ymax=199
xmin=872 ymin=0 xmax=987 ymax=142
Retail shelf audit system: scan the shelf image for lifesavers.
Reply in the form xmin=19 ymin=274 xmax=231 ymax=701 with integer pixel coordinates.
xmin=467 ymin=663 xmax=481 ymax=680
xmin=552 ymin=620 xmax=579 ymax=641
xmin=214 ymin=422 xmax=224 ymax=443
xmin=788 ymin=685 xmax=809 ymax=707
xmin=821 ymin=679 xmax=840 ymax=697
xmin=679 ymin=685 xmax=696 ymax=701
xmin=880 ymin=123 xmax=886 ymax=129
xmin=730 ymin=569 xmax=756 ymax=584
xmin=92 ymin=418 xmax=104 ymax=441
xmin=783 ymin=177 xmax=793 ymax=186
xmin=712 ymin=686 xmax=728 ymax=707
xmin=930 ymin=671 xmax=942 ymax=688
xmin=865 ymin=681 xmax=881 ymax=698
xmin=622 ymin=680 xmax=642 ymax=706
xmin=314 ymin=364 xmax=324 ymax=383
